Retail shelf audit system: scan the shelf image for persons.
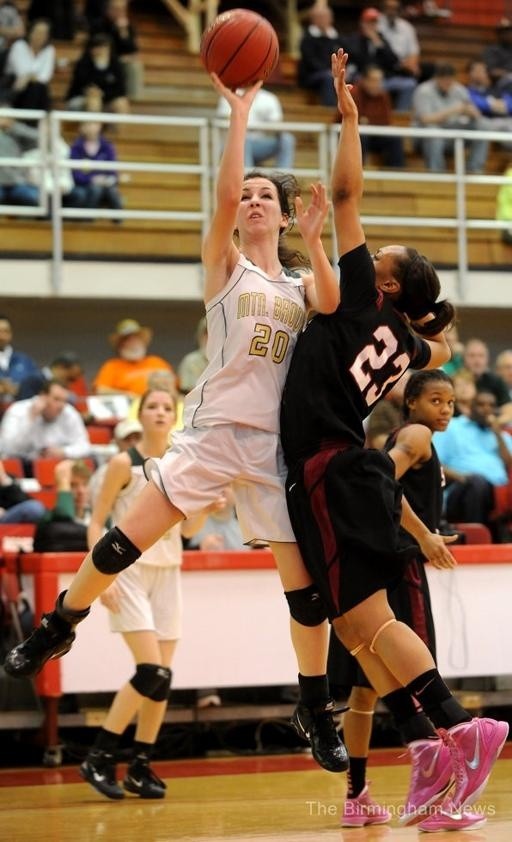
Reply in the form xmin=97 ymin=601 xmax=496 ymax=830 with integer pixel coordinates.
xmin=338 ymin=370 xmax=483 ymax=834
xmin=4 ymin=66 xmax=343 ymax=770
xmin=277 ymin=46 xmax=510 ymax=821
xmin=80 ymin=385 xmax=227 ymax=801
xmin=2 ymin=0 xmax=512 ymax=549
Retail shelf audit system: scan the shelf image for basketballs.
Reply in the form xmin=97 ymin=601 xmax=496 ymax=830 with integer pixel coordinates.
xmin=199 ymin=8 xmax=279 ymax=90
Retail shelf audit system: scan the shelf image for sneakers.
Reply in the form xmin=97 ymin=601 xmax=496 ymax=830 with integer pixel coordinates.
xmin=123 ymin=756 xmax=166 ymax=798
xmin=5 ymin=611 xmax=75 ymax=680
xmin=81 ymin=746 xmax=124 ymax=799
xmin=291 ymin=701 xmax=509 ymax=833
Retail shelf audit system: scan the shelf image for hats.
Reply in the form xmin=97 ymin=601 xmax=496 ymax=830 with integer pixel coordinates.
xmin=109 ymin=319 xmax=152 ymax=350
